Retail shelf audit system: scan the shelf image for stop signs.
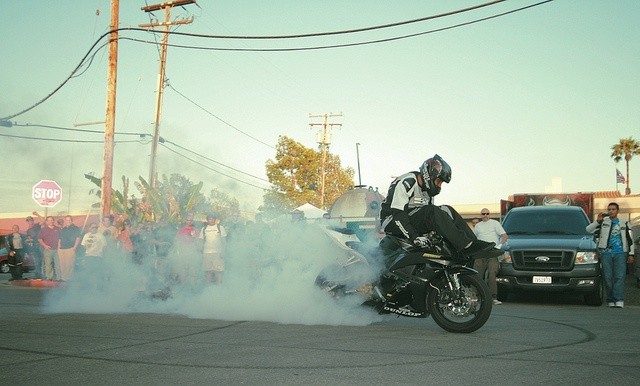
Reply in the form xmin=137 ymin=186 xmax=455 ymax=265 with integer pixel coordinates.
xmin=32 ymin=180 xmax=62 ymax=208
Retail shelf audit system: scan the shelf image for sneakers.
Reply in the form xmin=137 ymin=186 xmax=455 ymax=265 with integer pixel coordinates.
xmin=608 ymin=302 xmax=615 ymax=307
xmin=479 ymin=248 xmax=505 ymax=257
xmin=463 ymin=240 xmax=496 ymax=257
xmin=493 ymin=299 xmax=501 ymax=304
xmin=616 ymin=301 xmax=624 ymax=308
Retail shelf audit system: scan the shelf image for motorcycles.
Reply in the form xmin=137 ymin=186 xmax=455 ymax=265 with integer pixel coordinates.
xmin=316 ymin=226 xmax=492 ymax=333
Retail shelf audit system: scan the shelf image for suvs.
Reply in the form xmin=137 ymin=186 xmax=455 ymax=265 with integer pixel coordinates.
xmin=495 ymin=205 xmax=604 ymax=305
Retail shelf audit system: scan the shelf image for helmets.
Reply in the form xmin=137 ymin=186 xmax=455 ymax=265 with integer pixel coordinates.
xmin=419 ymin=154 xmax=451 ymax=197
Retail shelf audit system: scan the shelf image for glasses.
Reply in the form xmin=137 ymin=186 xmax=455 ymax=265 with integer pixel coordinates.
xmin=482 ymin=213 xmax=490 ymax=215
xmin=608 ymin=208 xmax=617 ymax=210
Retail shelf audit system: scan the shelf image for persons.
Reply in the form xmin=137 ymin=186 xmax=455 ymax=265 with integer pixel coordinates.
xmin=22 ymin=216 xmax=44 ymax=280
xmin=172 ymin=216 xmax=196 ymax=280
xmin=80 ymin=222 xmax=103 ymax=279
xmin=197 ymin=216 xmax=226 ymax=278
xmin=587 ymin=203 xmax=635 ymax=310
xmin=58 ymin=215 xmax=80 ymax=276
xmin=97 ymin=215 xmax=118 ymax=238
xmin=6 ymin=224 xmax=26 ymax=281
xmin=473 ymin=208 xmax=508 ymax=305
xmin=107 ymin=221 xmax=131 ymax=280
xmin=36 ymin=216 xmax=61 ymax=276
xmin=380 ymin=154 xmax=505 ymax=258
